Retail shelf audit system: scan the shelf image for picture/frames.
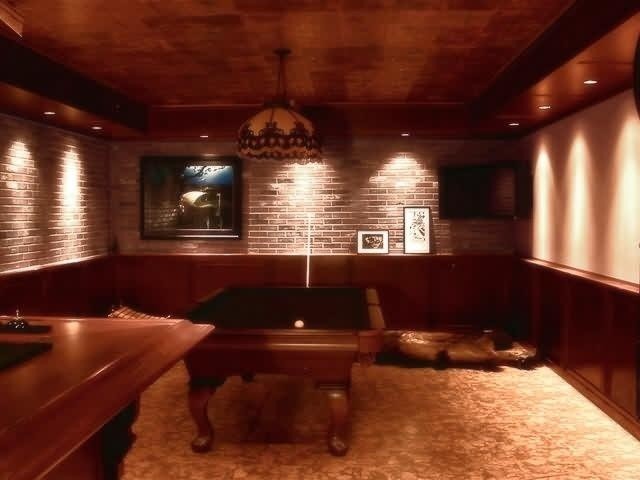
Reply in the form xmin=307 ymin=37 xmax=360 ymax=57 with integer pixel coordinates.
xmin=402 ymin=205 xmax=431 ymax=255
xmin=356 ymin=228 xmax=389 ymax=255
xmin=138 ymin=154 xmax=244 ymax=242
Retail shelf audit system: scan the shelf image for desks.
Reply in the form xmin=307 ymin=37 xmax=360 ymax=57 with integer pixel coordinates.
xmin=0 ymin=314 xmax=218 ymax=480
xmin=168 ymin=281 xmax=387 ymax=458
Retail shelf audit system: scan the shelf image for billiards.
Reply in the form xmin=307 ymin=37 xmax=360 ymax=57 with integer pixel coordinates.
xmin=295 ymin=321 xmax=304 ymax=328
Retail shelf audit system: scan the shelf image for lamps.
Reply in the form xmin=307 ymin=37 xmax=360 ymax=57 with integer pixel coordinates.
xmin=233 ymin=48 xmax=325 ymax=164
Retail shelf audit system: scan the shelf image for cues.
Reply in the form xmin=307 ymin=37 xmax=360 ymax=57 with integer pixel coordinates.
xmin=305 ymin=217 xmax=311 ymax=288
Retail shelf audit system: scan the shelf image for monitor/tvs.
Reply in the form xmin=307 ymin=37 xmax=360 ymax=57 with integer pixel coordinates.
xmin=435 ymin=159 xmax=528 ymax=220
